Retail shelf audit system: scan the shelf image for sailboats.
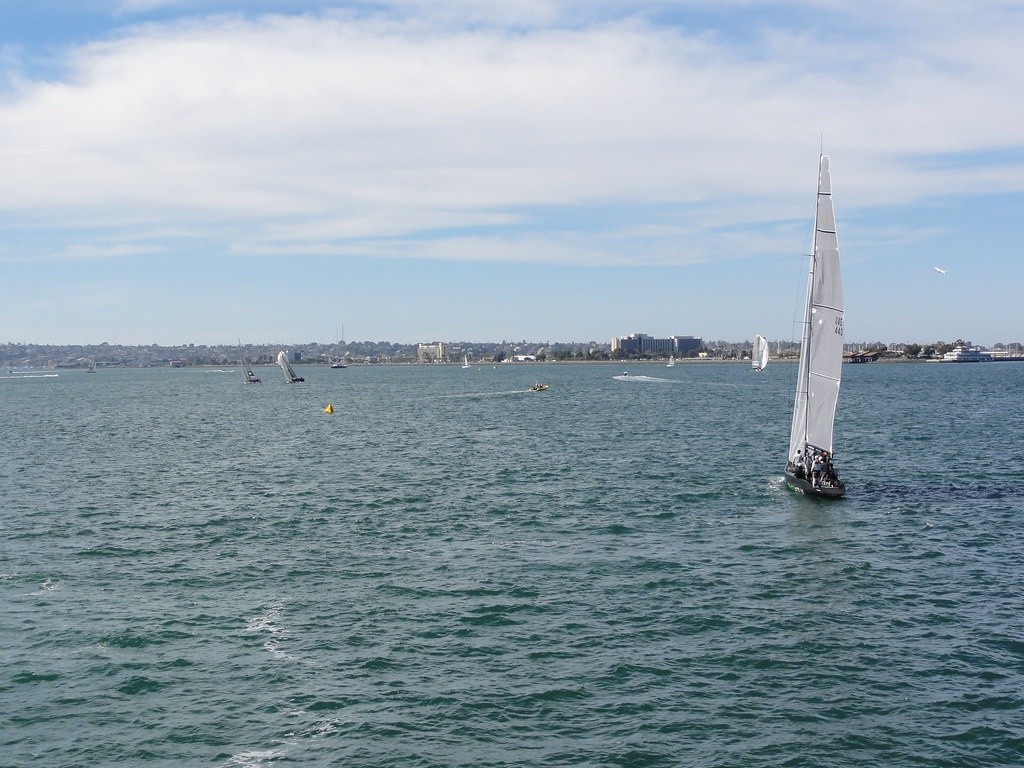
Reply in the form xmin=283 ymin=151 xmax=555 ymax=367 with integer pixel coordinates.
xmin=751 ymin=335 xmax=769 ymax=372
xmin=276 ymin=346 xmax=305 ymax=383
xmin=331 ymin=323 xmax=348 ymax=368
xmin=784 ymin=134 xmax=847 ymax=501
xmin=238 ymin=336 xmax=262 ymax=384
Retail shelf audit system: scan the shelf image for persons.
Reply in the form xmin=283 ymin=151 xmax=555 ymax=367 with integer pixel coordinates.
xmin=792 ymin=450 xmax=821 ymax=489
xmin=530 ymin=382 xmax=543 ymax=391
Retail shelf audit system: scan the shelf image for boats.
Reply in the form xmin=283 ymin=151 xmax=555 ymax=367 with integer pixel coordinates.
xmin=528 ymin=384 xmax=549 ymax=391
